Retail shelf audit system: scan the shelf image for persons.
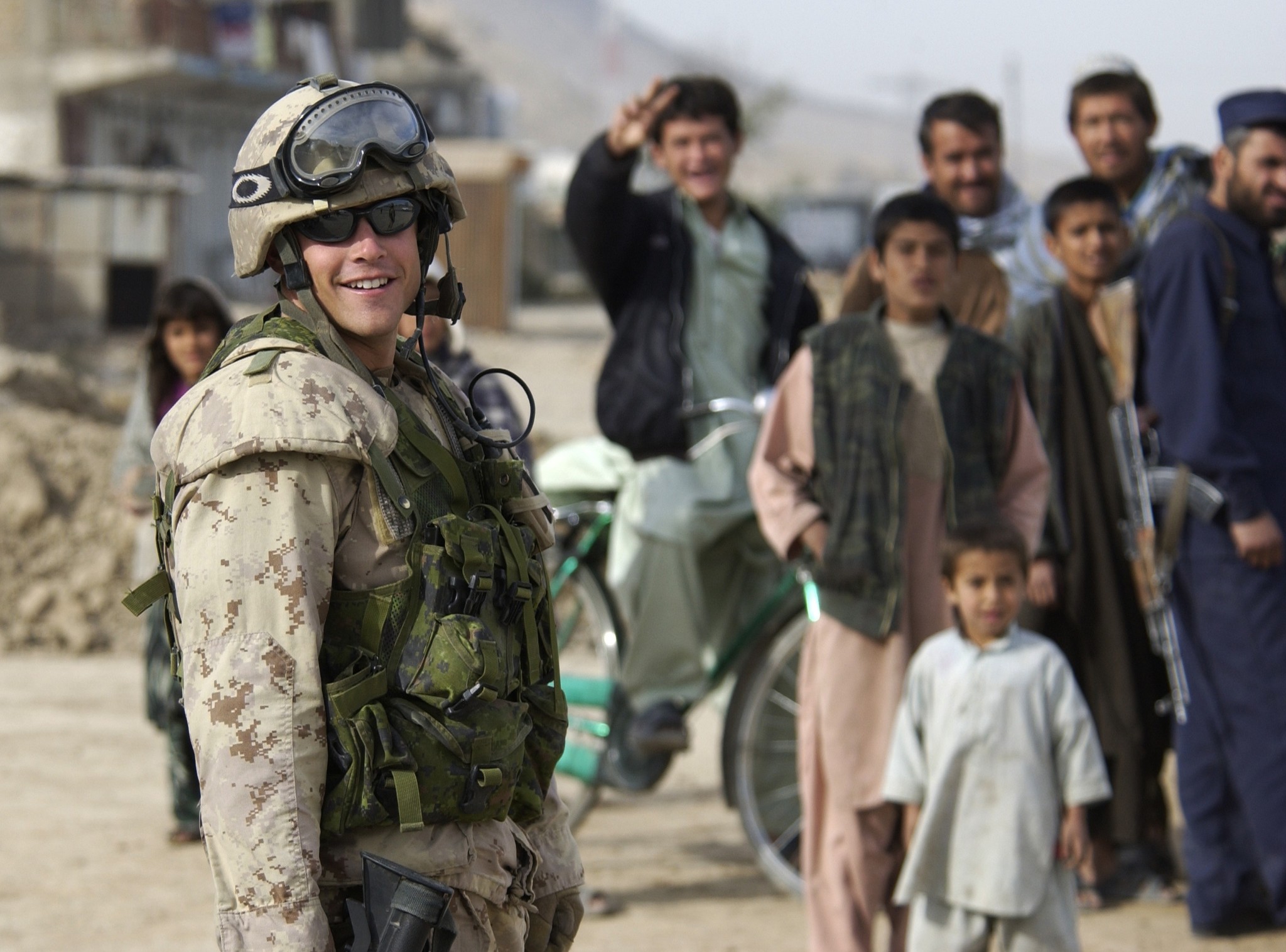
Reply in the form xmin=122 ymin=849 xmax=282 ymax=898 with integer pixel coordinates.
xmin=880 ymin=509 xmax=1105 ymax=952
xmin=1137 ymin=57 xmax=1285 ymax=940
xmin=112 ymin=271 xmax=243 ymax=842
xmin=744 ymin=192 xmax=1050 ymax=951
xmin=834 ymin=91 xmax=1068 ymax=422
xmin=563 ymin=76 xmax=834 ymax=790
xmin=345 ymin=227 xmax=537 ymax=690
xmin=121 ymin=72 xmax=586 ymax=952
xmin=1005 ymin=176 xmax=1192 ymax=911
xmin=1020 ymin=69 xmax=1225 ymax=901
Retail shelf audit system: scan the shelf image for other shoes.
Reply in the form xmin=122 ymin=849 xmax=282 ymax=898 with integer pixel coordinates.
xmin=169 ymin=825 xmax=200 ymax=844
xmin=1079 ymin=888 xmax=1108 ymax=913
xmin=1130 ymin=874 xmax=1181 ymax=905
xmin=605 ymin=699 xmax=682 ymax=794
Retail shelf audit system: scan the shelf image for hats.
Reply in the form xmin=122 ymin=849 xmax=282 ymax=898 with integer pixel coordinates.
xmin=1219 ymin=89 xmax=1286 ymax=139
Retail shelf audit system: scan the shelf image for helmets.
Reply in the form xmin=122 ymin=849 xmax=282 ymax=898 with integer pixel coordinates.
xmin=229 ymin=76 xmax=468 ymax=280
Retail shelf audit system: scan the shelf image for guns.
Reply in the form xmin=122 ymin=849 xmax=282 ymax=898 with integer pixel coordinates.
xmin=336 ymin=848 xmax=457 ymax=952
xmin=1089 ymin=273 xmax=1230 ymax=730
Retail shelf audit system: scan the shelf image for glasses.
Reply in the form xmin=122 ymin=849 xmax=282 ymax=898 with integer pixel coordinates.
xmin=293 ymin=195 xmax=419 ymax=243
xmin=277 ymin=78 xmax=430 ymax=199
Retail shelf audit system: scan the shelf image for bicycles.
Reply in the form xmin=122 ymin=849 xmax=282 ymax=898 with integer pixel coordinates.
xmin=533 ymin=386 xmax=810 ymax=904
